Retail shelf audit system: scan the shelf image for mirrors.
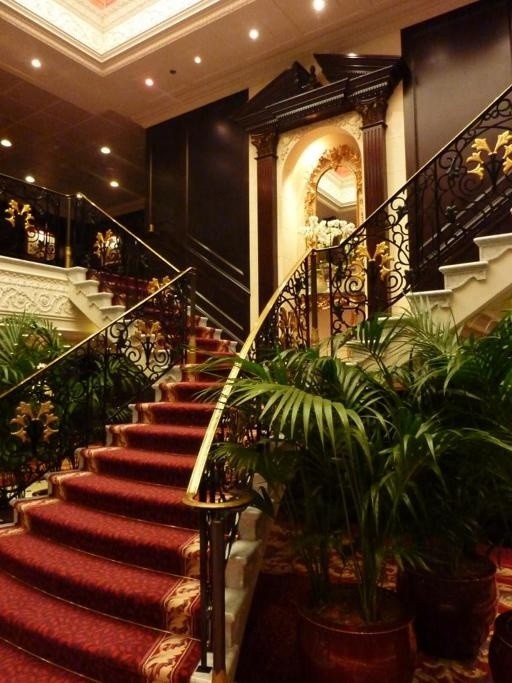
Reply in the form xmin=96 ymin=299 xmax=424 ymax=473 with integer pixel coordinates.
xmin=302 ymin=141 xmax=364 ymax=279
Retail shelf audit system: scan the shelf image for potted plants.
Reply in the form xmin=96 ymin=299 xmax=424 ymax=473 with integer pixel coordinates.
xmin=215 ymin=293 xmax=512 ymax=683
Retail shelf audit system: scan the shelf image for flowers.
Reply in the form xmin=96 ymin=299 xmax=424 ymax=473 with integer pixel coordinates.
xmin=304 ymin=214 xmax=358 ymax=264
